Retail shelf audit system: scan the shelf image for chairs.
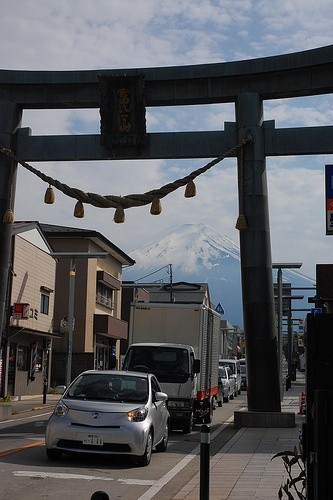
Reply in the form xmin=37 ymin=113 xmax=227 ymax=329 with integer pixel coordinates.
xmin=124 ymin=378 xmax=148 ymax=399
xmin=111 ymin=379 xmax=123 ymax=393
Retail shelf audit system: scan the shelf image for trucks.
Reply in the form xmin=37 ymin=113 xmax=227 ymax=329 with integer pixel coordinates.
xmin=108 ymin=302 xmax=220 ymax=433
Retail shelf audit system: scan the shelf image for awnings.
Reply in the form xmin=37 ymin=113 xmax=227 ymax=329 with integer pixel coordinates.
xmin=6 ymin=325 xmax=63 ymax=341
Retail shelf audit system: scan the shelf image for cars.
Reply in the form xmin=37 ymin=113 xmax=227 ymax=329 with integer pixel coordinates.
xmin=45 ymin=370 xmax=170 ymax=465
xmin=219 ymin=358 xmax=247 ymax=407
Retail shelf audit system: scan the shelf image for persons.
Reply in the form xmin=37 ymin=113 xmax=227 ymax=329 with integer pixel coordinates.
xmin=124 ymin=379 xmax=147 ymax=398
